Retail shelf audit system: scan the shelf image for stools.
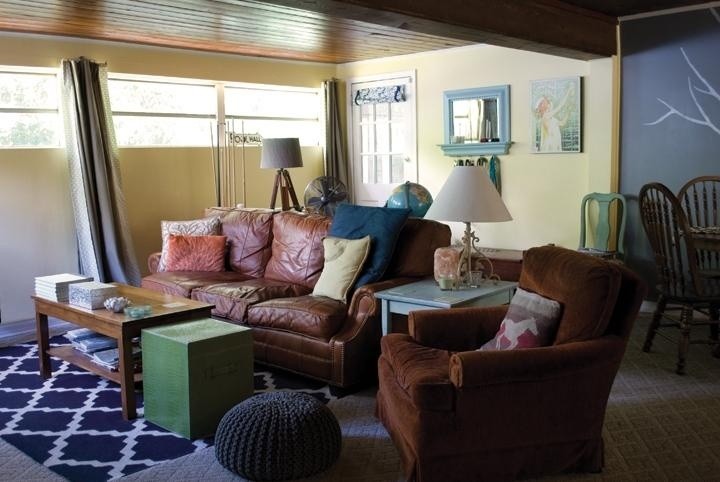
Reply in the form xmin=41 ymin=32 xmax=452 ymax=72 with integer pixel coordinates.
xmin=215 ymin=389 xmax=342 ymax=481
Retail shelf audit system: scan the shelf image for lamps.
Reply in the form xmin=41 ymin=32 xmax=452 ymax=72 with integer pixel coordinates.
xmin=422 ymin=165 xmax=516 ymax=290
xmin=260 ymin=138 xmax=303 ymax=212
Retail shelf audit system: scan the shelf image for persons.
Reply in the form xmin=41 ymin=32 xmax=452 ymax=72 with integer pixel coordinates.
xmin=535 ymin=89 xmax=570 ymax=152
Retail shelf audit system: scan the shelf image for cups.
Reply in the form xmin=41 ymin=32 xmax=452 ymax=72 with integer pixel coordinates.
xmin=439 ymin=272 xmax=455 ymax=290
xmin=468 ymin=271 xmax=483 ymax=288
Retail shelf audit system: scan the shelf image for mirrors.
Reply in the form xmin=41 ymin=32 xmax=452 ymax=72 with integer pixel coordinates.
xmin=436 ymin=84 xmax=511 ymax=156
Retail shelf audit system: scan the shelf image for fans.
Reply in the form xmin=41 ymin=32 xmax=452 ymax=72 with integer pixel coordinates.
xmin=304 ymin=175 xmax=353 ymax=218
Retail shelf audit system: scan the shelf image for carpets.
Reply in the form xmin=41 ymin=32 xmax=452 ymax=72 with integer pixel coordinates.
xmin=1 ymin=333 xmax=354 ymax=481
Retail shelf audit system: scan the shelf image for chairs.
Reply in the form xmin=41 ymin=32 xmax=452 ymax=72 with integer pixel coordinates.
xmin=676 ymin=175 xmax=720 ymax=288
xmin=638 ymin=182 xmax=720 ymax=375
xmin=374 ymin=244 xmax=646 ymax=481
xmin=574 ymin=192 xmax=628 ymax=264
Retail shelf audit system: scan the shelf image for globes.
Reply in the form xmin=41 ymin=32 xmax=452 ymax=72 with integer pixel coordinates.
xmin=384 ymin=181 xmax=433 ymax=218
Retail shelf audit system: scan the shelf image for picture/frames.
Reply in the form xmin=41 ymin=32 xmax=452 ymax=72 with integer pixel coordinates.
xmin=528 ymin=75 xmax=583 ymax=154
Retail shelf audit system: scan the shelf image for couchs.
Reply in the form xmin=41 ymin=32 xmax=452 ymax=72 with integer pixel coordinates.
xmin=140 ymin=206 xmax=449 ymax=390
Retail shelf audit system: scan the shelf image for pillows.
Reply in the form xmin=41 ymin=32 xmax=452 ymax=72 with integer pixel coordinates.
xmin=476 ymin=287 xmax=563 ymax=352
xmin=521 ymin=246 xmax=621 ymax=347
xmin=157 ymin=202 xmax=412 ymax=304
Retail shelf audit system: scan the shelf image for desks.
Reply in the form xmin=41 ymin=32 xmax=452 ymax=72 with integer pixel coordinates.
xmin=681 ymin=225 xmax=720 ymax=251
xmin=470 ymin=245 xmax=522 ymax=281
xmin=374 ymin=276 xmax=520 ymax=339
xmin=141 ymin=317 xmax=253 ymax=440
xmin=471 ymin=248 xmax=525 ymax=282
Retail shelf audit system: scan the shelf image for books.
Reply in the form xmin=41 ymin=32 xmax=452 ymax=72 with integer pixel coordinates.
xmin=63 ymin=327 xmax=142 ymax=371
xmin=35 ymin=272 xmax=94 ymax=303
